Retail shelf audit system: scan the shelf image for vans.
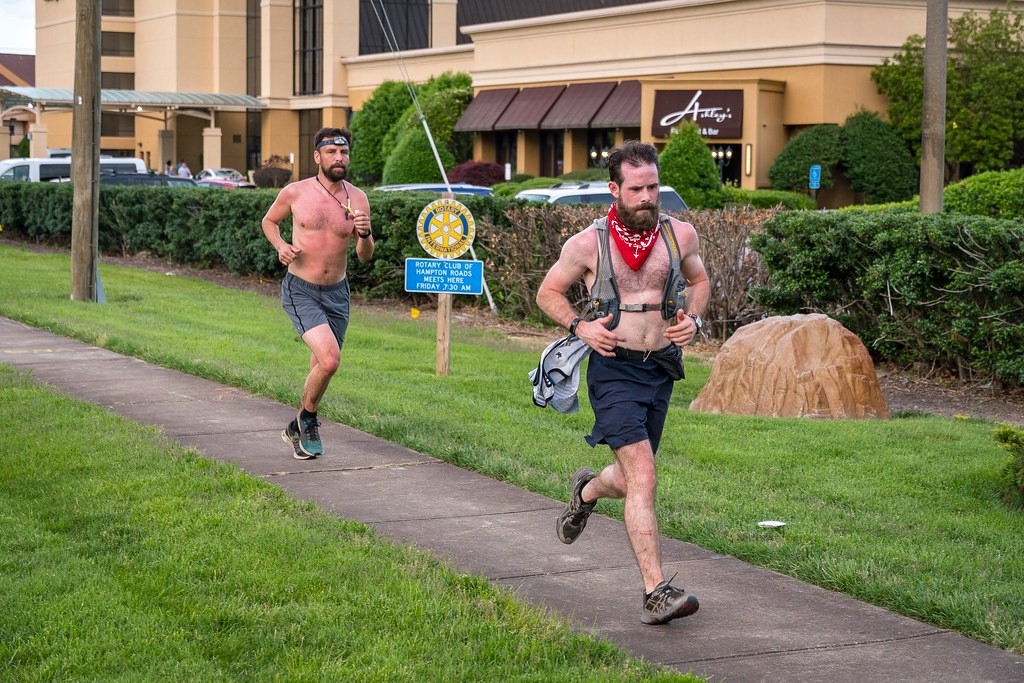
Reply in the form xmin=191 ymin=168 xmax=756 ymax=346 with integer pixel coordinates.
xmin=0 ymin=156 xmax=148 ymax=184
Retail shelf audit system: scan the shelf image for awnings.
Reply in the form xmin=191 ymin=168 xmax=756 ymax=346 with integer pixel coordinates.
xmin=453 ymin=78 xmax=641 ymax=128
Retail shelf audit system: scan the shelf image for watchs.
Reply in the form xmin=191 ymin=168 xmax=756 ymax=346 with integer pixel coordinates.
xmin=687 ymin=313 xmax=703 ymax=334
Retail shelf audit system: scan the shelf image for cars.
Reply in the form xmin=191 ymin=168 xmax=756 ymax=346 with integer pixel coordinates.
xmin=198 ymin=170 xmax=256 ymax=190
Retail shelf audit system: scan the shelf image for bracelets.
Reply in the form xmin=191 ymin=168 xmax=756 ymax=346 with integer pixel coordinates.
xmin=357 ymin=228 xmax=372 ymax=239
xmin=569 ymin=318 xmax=583 ymax=336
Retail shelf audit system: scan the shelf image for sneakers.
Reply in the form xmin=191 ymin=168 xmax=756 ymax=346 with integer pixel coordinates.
xmin=297 ymin=410 xmax=324 ymax=456
xmin=641 ymin=572 xmax=699 ymax=624
xmin=282 ymin=421 xmax=317 ymax=460
xmin=556 ymin=467 xmax=597 ymax=544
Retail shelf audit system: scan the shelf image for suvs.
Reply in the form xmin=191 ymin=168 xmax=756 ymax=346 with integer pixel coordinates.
xmin=513 ymin=183 xmax=690 ymax=217
xmin=97 ymin=175 xmax=199 ymax=187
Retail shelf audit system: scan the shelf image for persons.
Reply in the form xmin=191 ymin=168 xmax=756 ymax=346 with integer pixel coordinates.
xmin=165 ymin=160 xmax=171 ymax=175
xmin=177 ymin=162 xmax=193 ymax=179
xmin=176 ymin=159 xmax=184 ymax=171
xmin=534 ymin=140 xmax=713 ymax=624
xmin=261 ymin=129 xmax=375 ymax=460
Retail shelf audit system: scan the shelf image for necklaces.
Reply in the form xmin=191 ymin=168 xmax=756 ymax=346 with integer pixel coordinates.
xmin=316 ymin=174 xmax=356 ymax=217
